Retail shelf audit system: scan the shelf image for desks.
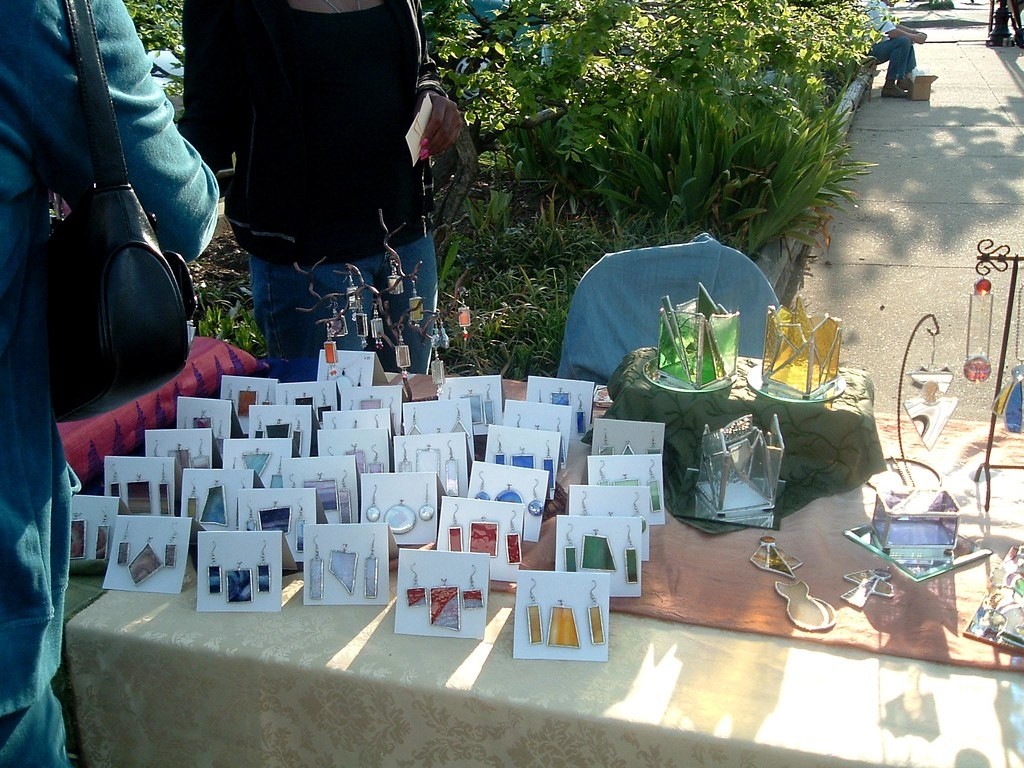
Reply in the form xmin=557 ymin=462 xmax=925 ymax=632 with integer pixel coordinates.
xmin=65 ymin=371 xmax=1024 ymax=767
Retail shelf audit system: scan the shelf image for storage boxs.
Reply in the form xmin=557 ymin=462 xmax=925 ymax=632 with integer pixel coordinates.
xmin=897 ymin=76 xmax=938 ymax=101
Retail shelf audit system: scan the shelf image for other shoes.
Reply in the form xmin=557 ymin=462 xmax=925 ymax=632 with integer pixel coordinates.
xmin=881 ymin=85 xmax=908 ymax=97
xmin=898 ymin=81 xmax=914 ymax=90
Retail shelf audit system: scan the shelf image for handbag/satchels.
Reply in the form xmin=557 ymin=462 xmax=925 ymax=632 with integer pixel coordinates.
xmin=39 ymin=0 xmax=200 ymax=423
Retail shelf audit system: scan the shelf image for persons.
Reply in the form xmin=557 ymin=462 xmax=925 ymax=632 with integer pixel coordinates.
xmin=862 ymin=0 xmax=928 ymax=98
xmin=179 ymin=0 xmax=465 ymax=375
xmin=1 ymin=0 xmax=221 ymax=768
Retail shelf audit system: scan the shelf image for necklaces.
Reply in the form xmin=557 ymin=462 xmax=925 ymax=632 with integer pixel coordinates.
xmin=323 ymin=0 xmax=362 ymax=13
